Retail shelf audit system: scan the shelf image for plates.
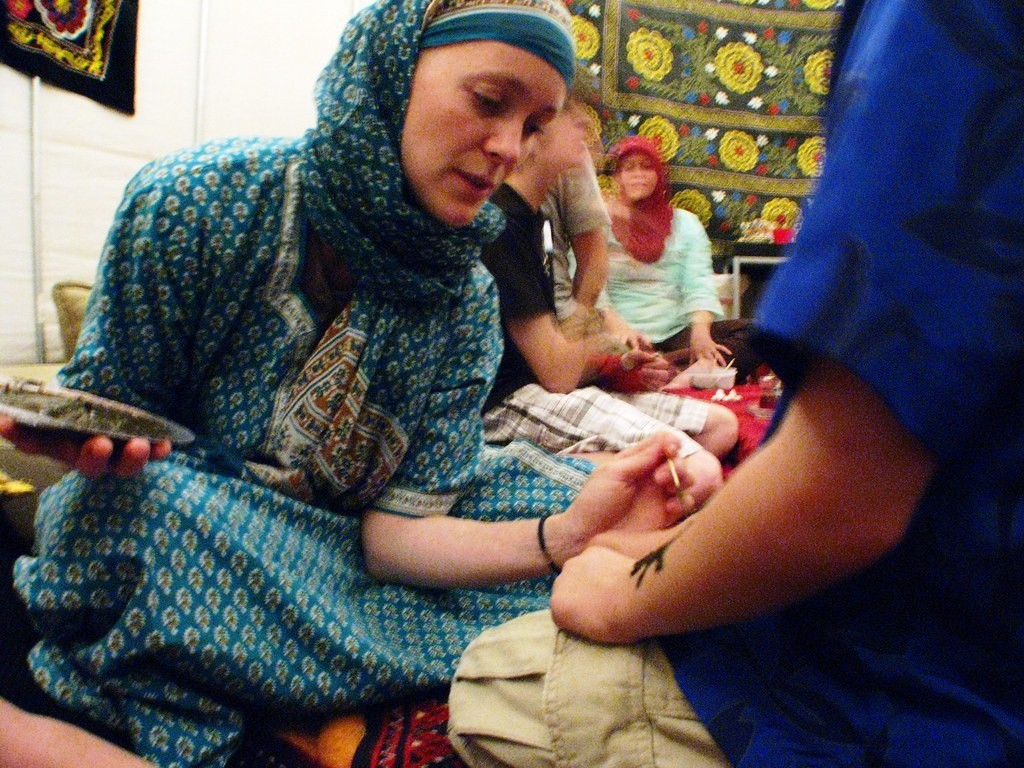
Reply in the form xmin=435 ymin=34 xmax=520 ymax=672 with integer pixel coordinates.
xmin=0 ymin=375 xmax=196 ymax=445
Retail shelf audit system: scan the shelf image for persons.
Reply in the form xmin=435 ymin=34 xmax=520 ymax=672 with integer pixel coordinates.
xmin=13 ymin=0 xmax=725 ymax=768
xmin=0 ymin=0 xmax=1024 ymax=768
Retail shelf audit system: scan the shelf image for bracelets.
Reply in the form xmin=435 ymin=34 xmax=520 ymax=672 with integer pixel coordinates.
xmin=538 ymin=509 xmax=561 ymax=574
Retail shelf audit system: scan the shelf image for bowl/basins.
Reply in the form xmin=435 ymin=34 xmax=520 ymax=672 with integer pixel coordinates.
xmin=689 ymin=366 xmax=738 ymax=390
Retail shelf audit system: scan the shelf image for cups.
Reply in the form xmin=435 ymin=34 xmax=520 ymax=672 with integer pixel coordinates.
xmin=773 ymin=228 xmax=794 ymax=245
